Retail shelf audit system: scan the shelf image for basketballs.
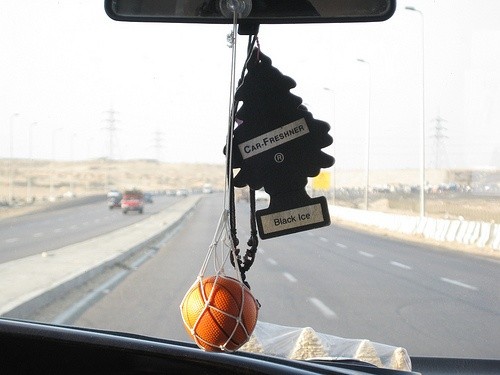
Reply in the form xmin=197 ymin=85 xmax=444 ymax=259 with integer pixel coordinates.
xmin=181 ymin=275 xmax=259 ymax=352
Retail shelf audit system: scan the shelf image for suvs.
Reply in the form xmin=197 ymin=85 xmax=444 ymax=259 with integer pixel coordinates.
xmin=108 ymin=188 xmax=152 ymax=214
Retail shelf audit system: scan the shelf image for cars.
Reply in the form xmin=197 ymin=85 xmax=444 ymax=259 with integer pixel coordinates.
xmin=166 ymin=187 xmax=211 ymax=198
